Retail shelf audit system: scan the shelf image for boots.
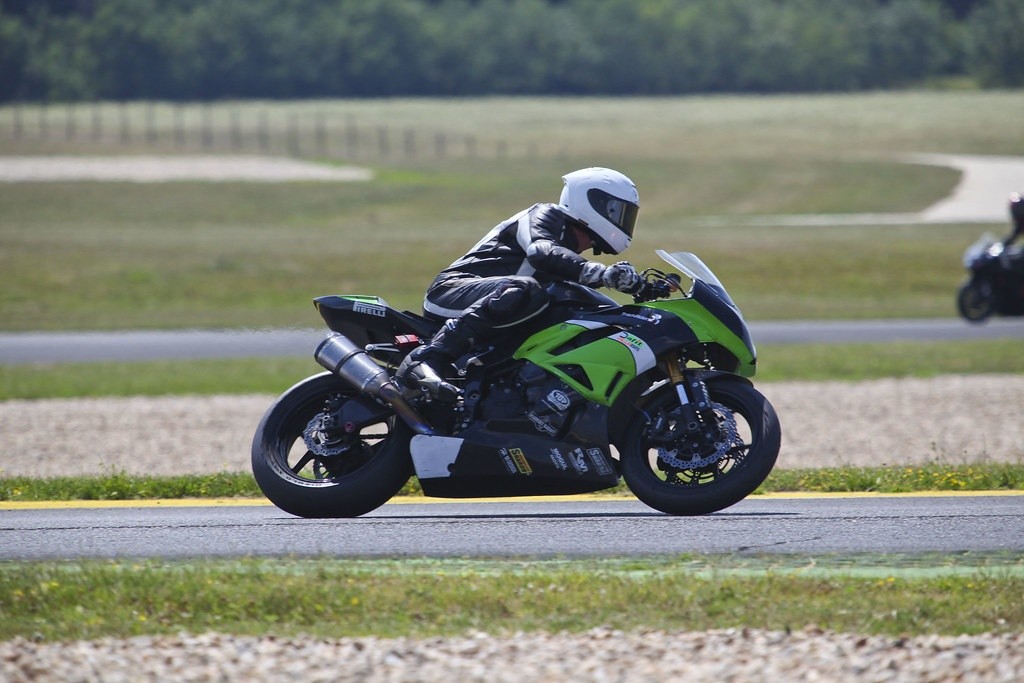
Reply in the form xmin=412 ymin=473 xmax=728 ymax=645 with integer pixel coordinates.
xmin=395 ymin=317 xmax=460 ymax=405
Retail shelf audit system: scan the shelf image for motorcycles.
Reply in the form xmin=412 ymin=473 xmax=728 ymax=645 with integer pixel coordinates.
xmin=956 ymin=189 xmax=1024 ymax=323
xmin=251 ymin=247 xmax=785 ymax=518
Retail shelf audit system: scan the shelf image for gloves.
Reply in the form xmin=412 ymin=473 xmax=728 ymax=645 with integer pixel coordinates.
xmin=579 ymin=260 xmax=643 ymax=293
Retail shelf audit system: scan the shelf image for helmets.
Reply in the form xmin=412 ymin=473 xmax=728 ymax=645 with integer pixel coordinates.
xmin=557 ymin=165 xmax=640 ymax=256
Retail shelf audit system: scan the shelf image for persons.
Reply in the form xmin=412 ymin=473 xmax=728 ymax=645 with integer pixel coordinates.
xmin=1001 ymin=193 xmax=1024 ymax=247
xmin=394 ymin=167 xmax=643 ymax=405
